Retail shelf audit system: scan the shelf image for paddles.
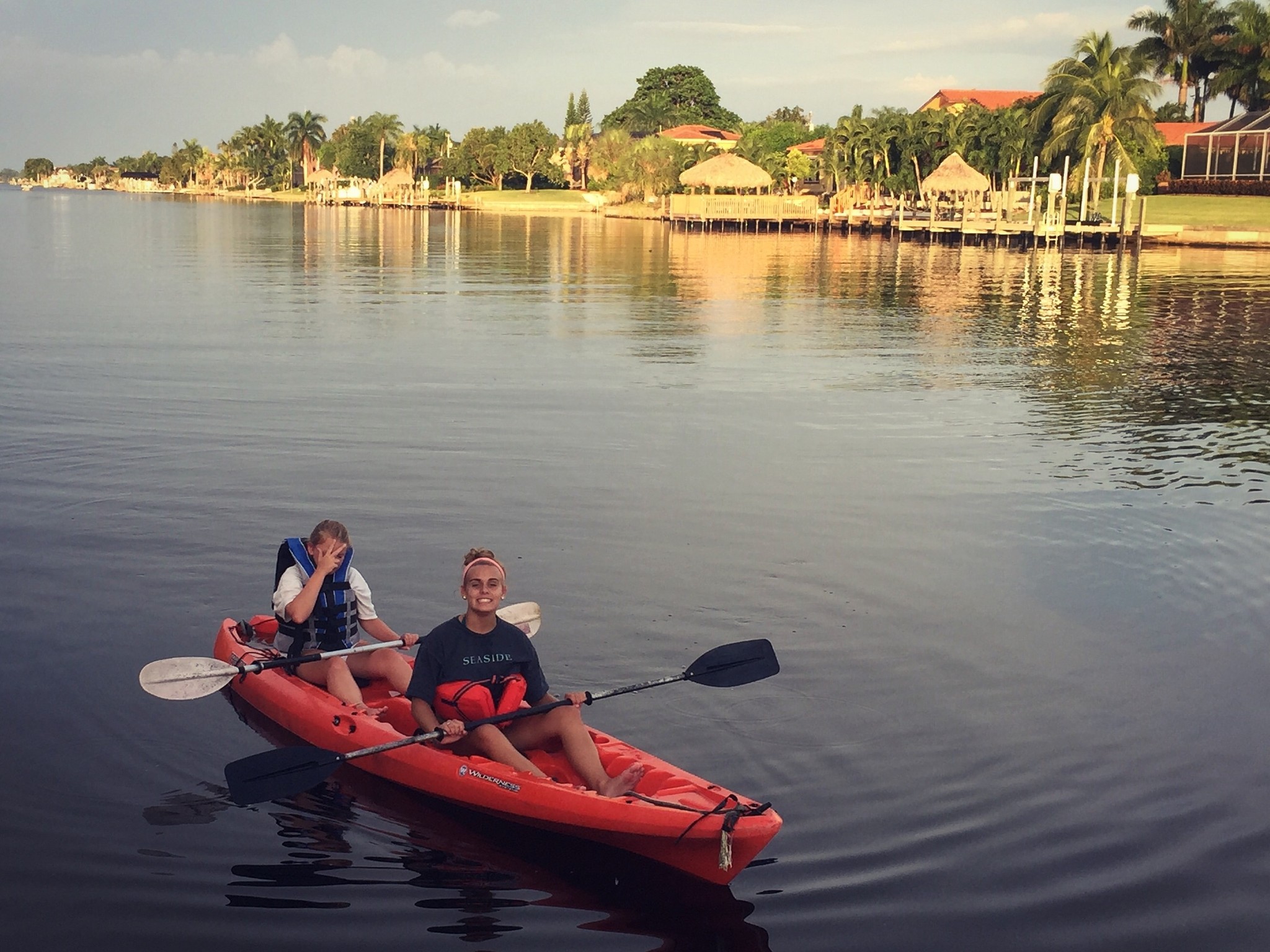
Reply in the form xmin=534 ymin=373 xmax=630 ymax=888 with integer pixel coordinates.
xmin=139 ymin=601 xmax=542 ymax=700
xmin=226 ymin=638 xmax=780 ymax=806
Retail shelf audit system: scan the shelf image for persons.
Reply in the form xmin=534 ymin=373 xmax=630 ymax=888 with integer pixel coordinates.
xmin=271 ymin=519 xmax=419 ymax=716
xmin=404 ymin=549 xmax=645 ymax=798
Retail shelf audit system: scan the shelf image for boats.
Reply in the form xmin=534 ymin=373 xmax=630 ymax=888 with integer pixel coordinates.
xmin=213 ymin=615 xmax=791 ymax=888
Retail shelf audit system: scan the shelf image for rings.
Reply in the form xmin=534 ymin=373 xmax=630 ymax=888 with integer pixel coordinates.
xmin=447 ymin=722 xmax=450 ymax=725
